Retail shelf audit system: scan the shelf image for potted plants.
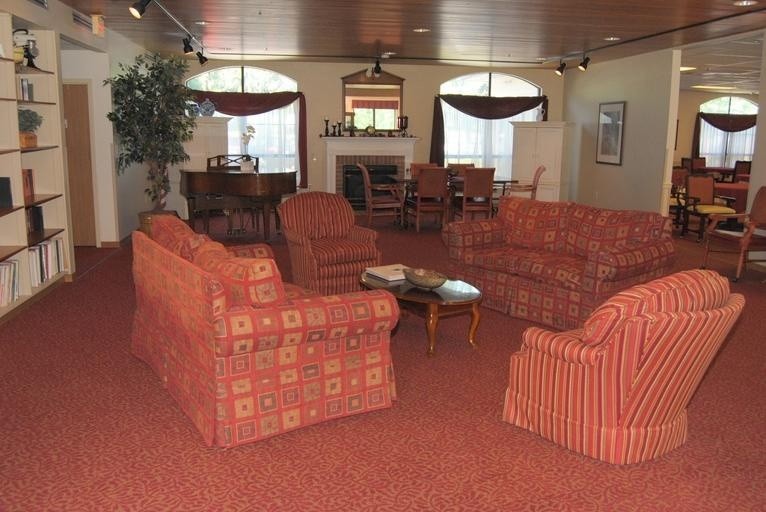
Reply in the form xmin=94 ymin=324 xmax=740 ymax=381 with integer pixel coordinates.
xmin=103 ymin=52 xmax=199 ymax=236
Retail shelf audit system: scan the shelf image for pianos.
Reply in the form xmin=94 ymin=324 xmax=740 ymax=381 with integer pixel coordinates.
xmin=179 ymin=154 xmax=296 ymax=241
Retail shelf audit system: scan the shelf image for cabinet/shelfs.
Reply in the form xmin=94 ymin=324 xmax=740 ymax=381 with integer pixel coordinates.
xmin=0 ymin=8 xmax=76 ymax=325
xmin=509 ymin=121 xmax=575 ymax=202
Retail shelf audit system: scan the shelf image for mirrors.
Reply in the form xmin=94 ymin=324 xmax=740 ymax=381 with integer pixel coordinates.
xmin=340 ymin=68 xmax=406 ymax=131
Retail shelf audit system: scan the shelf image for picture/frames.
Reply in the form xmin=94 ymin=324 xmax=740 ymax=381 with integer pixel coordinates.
xmin=596 ymin=100 xmax=625 ymax=166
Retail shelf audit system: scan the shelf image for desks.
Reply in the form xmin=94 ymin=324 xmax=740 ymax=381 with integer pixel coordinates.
xmin=321 ymin=135 xmax=422 ymax=197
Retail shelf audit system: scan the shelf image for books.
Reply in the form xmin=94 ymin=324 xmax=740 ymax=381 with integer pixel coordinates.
xmin=25 ymin=206 xmax=44 ymax=235
xmin=29 ymin=236 xmax=65 ymax=288
xmin=0 ymin=260 xmax=19 ymax=308
xmin=16 ymin=76 xmax=34 ymax=101
xmin=365 ymin=263 xmax=411 ymax=281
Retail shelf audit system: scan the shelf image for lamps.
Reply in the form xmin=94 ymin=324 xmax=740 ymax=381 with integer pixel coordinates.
xmin=555 ymin=61 xmax=566 ymax=77
xmin=373 ymin=57 xmax=382 ymax=79
xmin=129 ymin=0 xmax=208 ymax=67
xmin=578 ymin=57 xmax=590 ymax=70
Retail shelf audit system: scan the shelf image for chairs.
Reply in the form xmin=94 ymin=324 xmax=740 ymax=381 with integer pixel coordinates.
xmin=504 ymin=164 xmax=546 ymax=201
xmin=672 ymin=156 xmax=752 ymax=243
xmin=356 ymin=163 xmax=404 ymax=229
xmin=276 ymin=191 xmax=382 ymax=295
xmin=404 ymin=163 xmax=495 ymax=232
xmin=702 ymin=186 xmax=766 ymax=283
xmin=500 ymin=268 xmax=746 ymax=465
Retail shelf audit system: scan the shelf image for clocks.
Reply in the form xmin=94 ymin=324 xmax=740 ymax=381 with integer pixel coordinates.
xmin=200 ymin=99 xmax=215 ymax=116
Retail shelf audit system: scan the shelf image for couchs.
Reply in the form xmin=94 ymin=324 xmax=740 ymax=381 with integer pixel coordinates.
xmin=130 ymin=214 xmax=401 ymax=448
xmin=436 ymin=195 xmax=676 ymax=332
